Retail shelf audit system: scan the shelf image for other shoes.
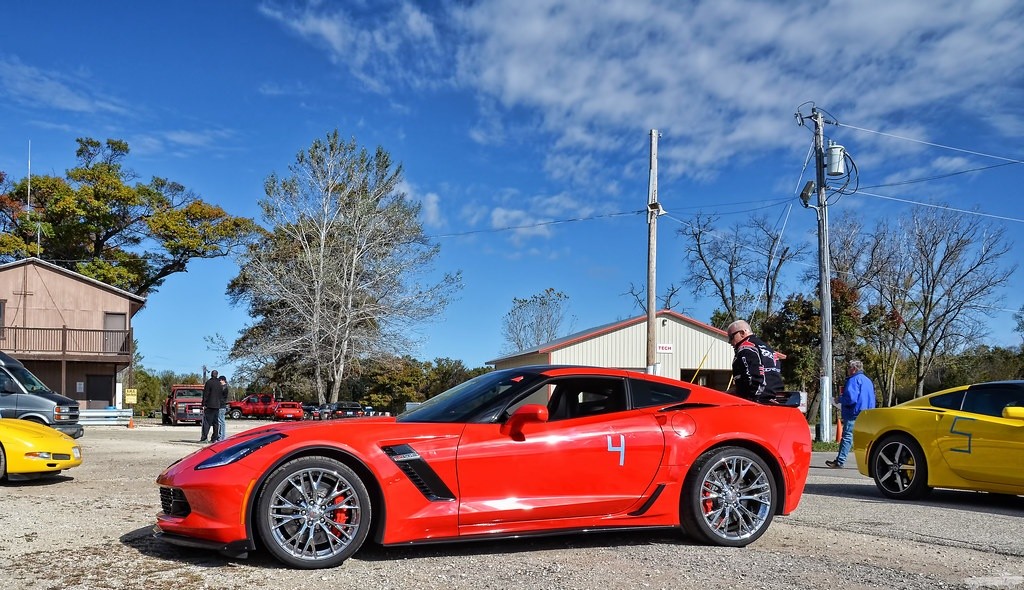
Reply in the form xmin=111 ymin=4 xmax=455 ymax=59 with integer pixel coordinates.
xmin=200 ymin=438 xmax=208 ymax=443
xmin=211 ymin=438 xmax=218 ymax=443
xmin=825 ymin=460 xmax=844 ymax=469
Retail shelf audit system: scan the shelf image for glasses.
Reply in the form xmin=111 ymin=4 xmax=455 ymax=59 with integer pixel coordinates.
xmin=729 ymin=330 xmax=746 ymax=341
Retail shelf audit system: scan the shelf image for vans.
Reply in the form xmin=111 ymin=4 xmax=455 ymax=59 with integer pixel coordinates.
xmin=0 ymin=350 xmax=84 ymax=439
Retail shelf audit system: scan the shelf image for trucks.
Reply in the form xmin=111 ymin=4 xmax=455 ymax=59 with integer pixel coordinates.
xmin=226 ymin=393 xmax=303 ymax=419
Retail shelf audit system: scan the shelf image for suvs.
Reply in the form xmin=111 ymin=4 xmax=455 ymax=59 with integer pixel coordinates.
xmin=162 ymin=384 xmax=206 ymax=425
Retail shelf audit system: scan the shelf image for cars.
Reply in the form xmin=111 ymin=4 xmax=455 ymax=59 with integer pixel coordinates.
xmin=272 ymin=401 xmax=304 ymax=422
xmin=402 ymin=402 xmax=423 ymax=413
xmin=301 ymin=400 xmax=374 ymax=421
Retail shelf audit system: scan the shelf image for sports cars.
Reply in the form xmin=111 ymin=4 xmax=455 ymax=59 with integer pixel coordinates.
xmin=0 ymin=417 xmax=83 ymax=486
xmin=853 ymin=380 xmax=1024 ymax=500
xmin=155 ymin=364 xmax=811 ymax=571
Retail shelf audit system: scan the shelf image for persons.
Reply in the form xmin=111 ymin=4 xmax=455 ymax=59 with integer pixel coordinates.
xmin=217 ymin=376 xmax=228 ymax=442
xmin=825 ymin=360 xmax=875 ymax=469
xmin=726 ymin=320 xmax=785 ymax=404
xmin=197 ymin=370 xmax=224 ymax=444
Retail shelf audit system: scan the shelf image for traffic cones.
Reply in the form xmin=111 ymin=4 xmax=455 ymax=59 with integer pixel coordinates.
xmin=833 ymin=418 xmax=843 ymax=443
xmin=128 ymin=417 xmax=135 ymax=428
